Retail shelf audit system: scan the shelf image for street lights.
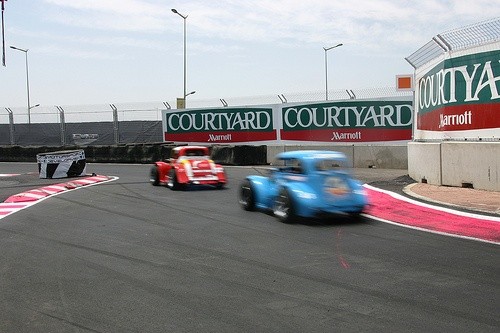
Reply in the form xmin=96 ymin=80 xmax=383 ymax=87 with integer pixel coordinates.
xmin=9 ymin=46 xmax=40 ymax=124
xmin=171 ymin=9 xmax=195 ymax=108
xmin=322 ymin=44 xmax=342 ymax=100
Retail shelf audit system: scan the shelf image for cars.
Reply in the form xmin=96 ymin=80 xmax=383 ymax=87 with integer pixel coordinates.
xmin=150 ymin=146 xmax=228 ymax=190
xmin=239 ymin=150 xmax=368 ymax=223
